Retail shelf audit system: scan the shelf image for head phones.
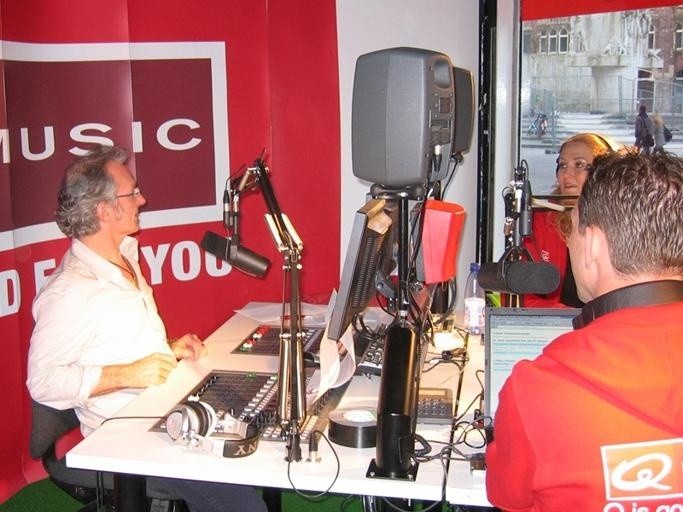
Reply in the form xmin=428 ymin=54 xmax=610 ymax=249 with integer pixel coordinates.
xmin=164 ymin=399 xmax=260 ymax=459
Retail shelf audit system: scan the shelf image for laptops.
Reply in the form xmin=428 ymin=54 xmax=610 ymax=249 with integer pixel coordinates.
xmin=483 ymin=306 xmax=581 ymax=441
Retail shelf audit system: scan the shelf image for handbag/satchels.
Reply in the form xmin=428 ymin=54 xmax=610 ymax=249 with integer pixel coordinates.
xmin=664 ymin=126 xmax=672 ymax=142
xmin=645 ymin=133 xmax=654 ymax=147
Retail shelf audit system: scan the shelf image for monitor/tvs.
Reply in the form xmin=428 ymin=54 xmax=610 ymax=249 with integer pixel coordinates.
xmin=326 ymin=198 xmax=400 ymax=341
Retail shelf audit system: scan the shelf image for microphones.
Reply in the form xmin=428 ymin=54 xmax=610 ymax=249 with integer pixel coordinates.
xmin=476 ymin=260 xmax=561 ymax=294
xmin=200 ymin=232 xmax=272 ymax=279
xmin=520 ymin=181 xmax=533 ymax=239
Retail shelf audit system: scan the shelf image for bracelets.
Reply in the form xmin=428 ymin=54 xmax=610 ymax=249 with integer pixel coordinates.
xmin=165 ymin=336 xmax=177 ymax=348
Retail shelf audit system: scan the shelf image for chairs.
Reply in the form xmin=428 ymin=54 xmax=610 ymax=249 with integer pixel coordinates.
xmin=26 ymin=381 xmax=286 ymax=511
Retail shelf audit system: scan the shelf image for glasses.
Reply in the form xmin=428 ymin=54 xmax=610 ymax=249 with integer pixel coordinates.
xmin=116 ymin=186 xmax=141 ymax=199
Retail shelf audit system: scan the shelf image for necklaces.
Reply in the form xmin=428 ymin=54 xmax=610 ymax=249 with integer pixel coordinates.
xmin=107 ymin=253 xmax=136 ymax=281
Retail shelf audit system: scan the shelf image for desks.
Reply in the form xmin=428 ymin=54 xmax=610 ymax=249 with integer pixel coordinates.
xmin=440 ymin=309 xmax=501 ymax=512
xmin=63 ymin=295 xmax=472 ymax=508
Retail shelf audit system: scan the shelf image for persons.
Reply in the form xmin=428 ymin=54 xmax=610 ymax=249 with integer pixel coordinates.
xmin=497 ymin=131 xmax=640 ymax=314
xmin=23 ymin=143 xmax=275 ymax=511
xmin=634 ymin=105 xmax=653 ymax=151
xmin=483 ymin=145 xmax=682 ymax=511
xmin=649 ymin=111 xmax=668 ymax=155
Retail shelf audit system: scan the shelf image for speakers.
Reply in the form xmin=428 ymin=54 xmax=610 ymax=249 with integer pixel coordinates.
xmin=353 ymin=48 xmax=452 ymax=188
xmin=453 ymin=66 xmax=475 ymax=161
